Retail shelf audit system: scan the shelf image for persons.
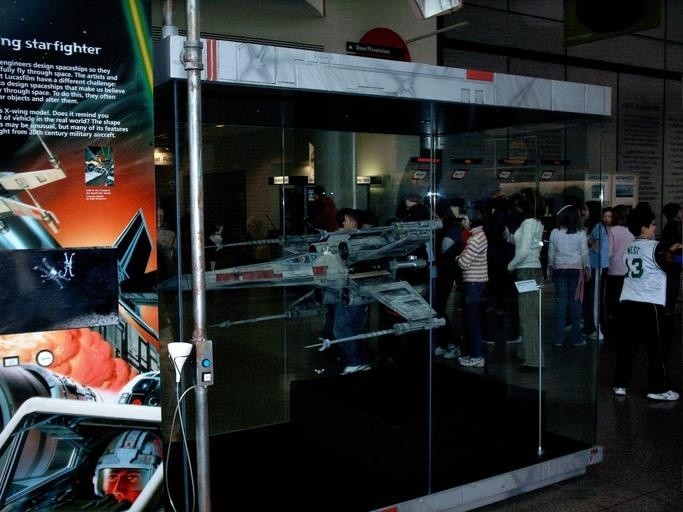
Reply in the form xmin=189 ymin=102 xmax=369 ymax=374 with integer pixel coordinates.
xmin=313 ymin=185 xmax=683 ymax=402
xmin=102 ymin=468 xmax=143 ymax=503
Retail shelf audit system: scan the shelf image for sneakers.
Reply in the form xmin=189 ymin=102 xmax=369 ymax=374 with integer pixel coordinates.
xmin=647 ymin=390 xmax=680 ymax=401
xmin=435 ymin=343 xmax=486 ymax=369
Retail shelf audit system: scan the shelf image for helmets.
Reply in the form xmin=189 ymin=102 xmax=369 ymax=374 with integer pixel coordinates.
xmin=92 ymin=430 xmax=166 ymax=501
